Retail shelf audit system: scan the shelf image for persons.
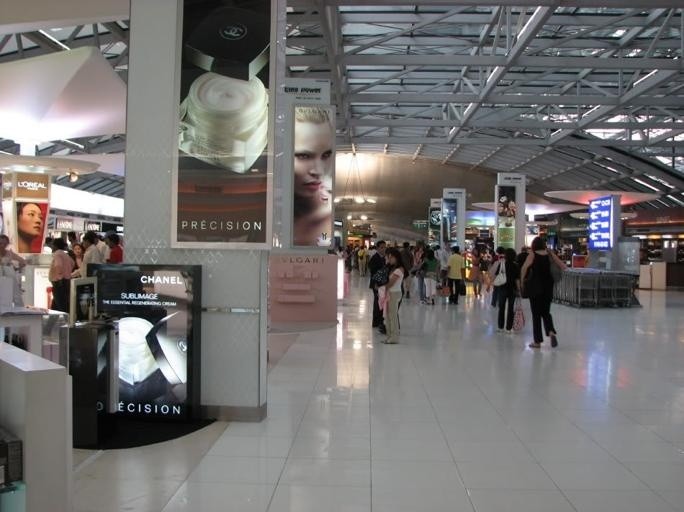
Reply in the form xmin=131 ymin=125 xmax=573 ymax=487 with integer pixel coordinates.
xmin=45 ymin=229 xmax=122 ymax=312
xmin=17 ymin=202 xmax=43 ymax=253
xmin=293 ymin=107 xmax=333 ymax=245
xmin=335 ymin=241 xmax=531 ymax=344
xmin=0 ymin=235 xmax=26 ymax=270
xmin=520 ymin=236 xmax=568 ymax=348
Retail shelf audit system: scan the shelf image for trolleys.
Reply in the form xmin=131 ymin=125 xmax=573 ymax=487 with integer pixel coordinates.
xmin=552 ymin=266 xmax=638 ymax=310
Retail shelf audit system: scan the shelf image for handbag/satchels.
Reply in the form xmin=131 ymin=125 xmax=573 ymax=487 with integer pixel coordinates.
xmin=512 ymin=309 xmax=525 ymax=330
xmin=550 ymin=261 xmax=560 ymax=281
xmin=459 ymin=284 xmax=466 ymax=295
xmin=442 ymin=287 xmax=450 ymax=296
xmin=491 ymin=273 xmax=507 ymax=287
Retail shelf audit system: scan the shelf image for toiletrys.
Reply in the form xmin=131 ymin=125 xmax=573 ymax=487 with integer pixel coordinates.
xmin=177 ymin=6 xmax=269 ymax=175
xmin=107 ymin=309 xmax=188 ymax=404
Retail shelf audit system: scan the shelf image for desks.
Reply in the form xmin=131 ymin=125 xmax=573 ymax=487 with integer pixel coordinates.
xmin=652 ymin=262 xmax=684 ymax=290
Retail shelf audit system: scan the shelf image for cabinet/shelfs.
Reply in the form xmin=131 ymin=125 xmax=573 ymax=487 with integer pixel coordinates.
xmin=639 ymin=264 xmax=651 ymax=288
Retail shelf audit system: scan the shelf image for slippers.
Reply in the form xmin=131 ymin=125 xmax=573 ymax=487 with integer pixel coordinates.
xmin=529 ymin=343 xmax=540 ymax=348
xmin=551 ymin=335 xmax=557 ymax=347
